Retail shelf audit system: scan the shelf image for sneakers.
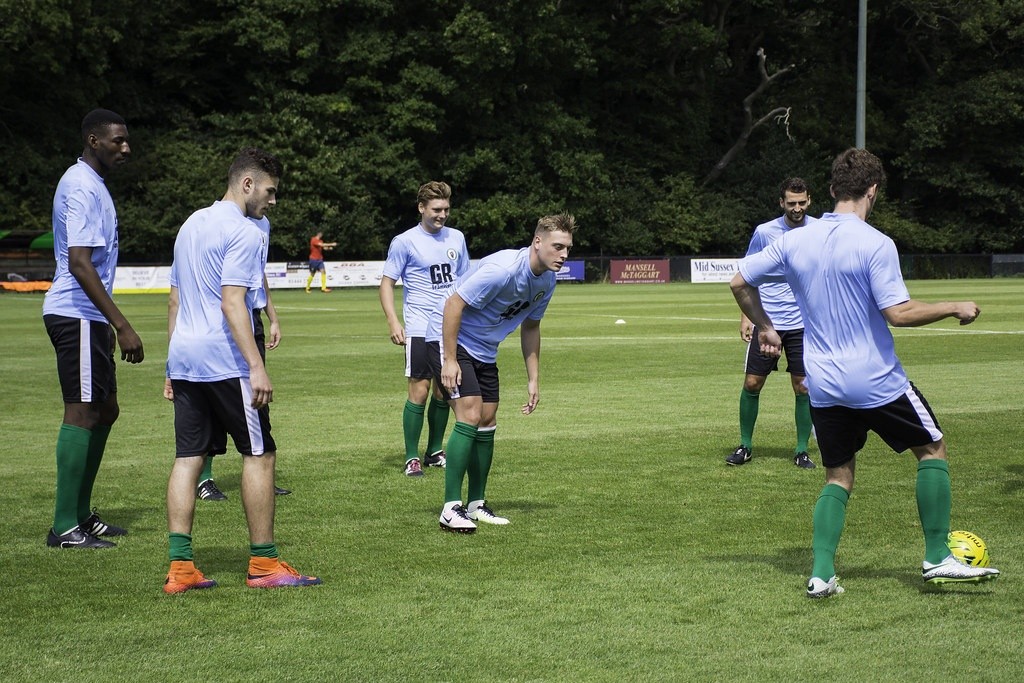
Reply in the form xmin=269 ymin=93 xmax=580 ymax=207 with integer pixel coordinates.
xmin=46 ymin=526 xmax=117 ymax=547
xmin=794 ymin=451 xmax=816 ymax=468
xmin=465 ymin=500 xmax=510 ymax=525
xmin=726 ymin=445 xmax=751 ymax=465
xmin=401 ymin=458 xmax=423 ymax=476
xmin=274 ymin=486 xmax=292 ymax=496
xmin=439 ymin=500 xmax=477 ymax=532
xmin=246 ymin=562 xmax=322 ymax=587
xmin=808 ymin=574 xmax=845 ymax=597
xmin=79 ymin=506 xmax=127 ymax=536
xmin=422 ymin=449 xmax=447 ymax=468
xmin=923 ymin=552 xmax=1000 ymax=584
xmin=163 ymin=569 xmax=218 ymax=592
xmin=195 ymin=479 xmax=228 ymax=500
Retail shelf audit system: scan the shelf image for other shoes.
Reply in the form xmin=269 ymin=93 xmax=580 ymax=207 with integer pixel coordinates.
xmin=306 ymin=287 xmax=311 ymax=293
xmin=322 ymin=289 xmax=331 ymax=292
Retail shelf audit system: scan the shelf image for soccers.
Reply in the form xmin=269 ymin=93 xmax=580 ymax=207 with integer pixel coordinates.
xmin=946 ymin=530 xmax=991 ymax=568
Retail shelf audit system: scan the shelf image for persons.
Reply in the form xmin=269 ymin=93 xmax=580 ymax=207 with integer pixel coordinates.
xmin=306 ymin=229 xmax=337 ymax=294
xmin=196 ymin=216 xmax=292 ymax=500
xmin=379 ymin=181 xmax=472 ymax=475
xmin=164 ymin=147 xmax=320 ymax=595
xmin=42 ymin=108 xmax=145 ymax=551
xmin=438 ymin=214 xmax=577 ymax=532
xmin=725 ymin=177 xmax=827 ymax=470
xmin=728 ymin=148 xmax=1001 ymax=598
